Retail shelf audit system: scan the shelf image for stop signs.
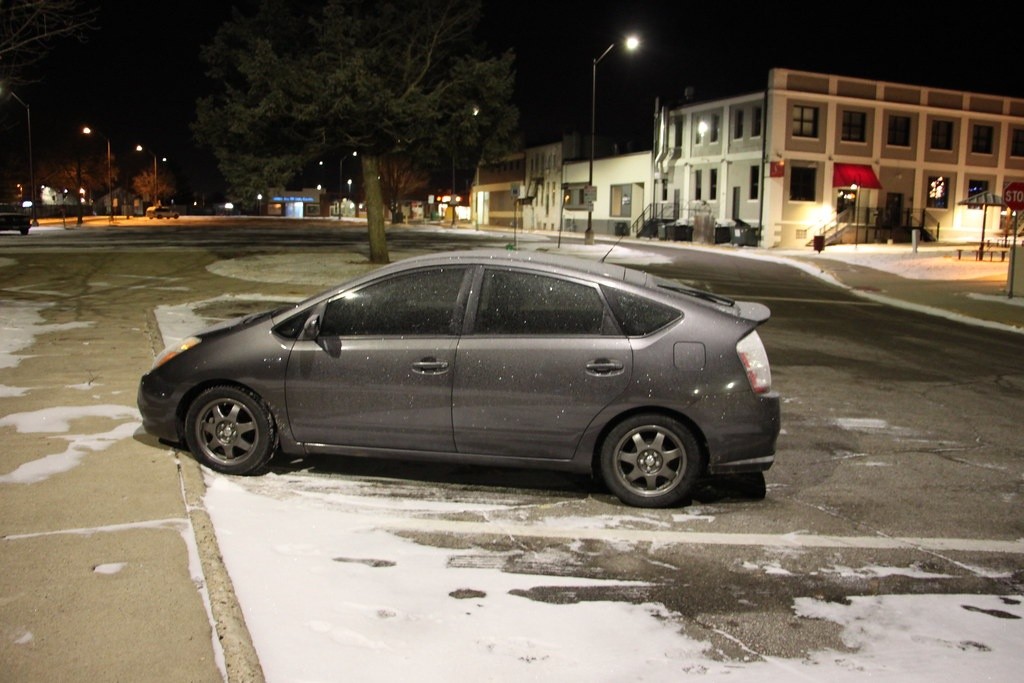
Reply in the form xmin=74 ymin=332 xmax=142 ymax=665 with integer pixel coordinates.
xmin=1002 ymin=180 xmax=1024 ymax=212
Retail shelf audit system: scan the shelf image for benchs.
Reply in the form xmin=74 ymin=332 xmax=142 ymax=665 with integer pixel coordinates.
xmin=957 ymin=249 xmax=1008 ymax=264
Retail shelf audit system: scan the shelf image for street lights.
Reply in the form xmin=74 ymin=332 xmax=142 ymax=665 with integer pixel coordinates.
xmin=83 ymin=127 xmax=114 ymax=223
xmin=584 ymin=33 xmax=640 ymax=246
xmin=136 ymin=145 xmax=157 ymax=205
xmin=451 ymin=105 xmax=478 ymax=230
xmin=338 ymin=151 xmax=357 ymax=219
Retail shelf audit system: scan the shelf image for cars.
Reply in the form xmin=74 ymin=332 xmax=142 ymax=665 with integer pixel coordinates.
xmin=136 ymin=247 xmax=782 ymax=509
xmin=146 ymin=201 xmax=180 ymax=220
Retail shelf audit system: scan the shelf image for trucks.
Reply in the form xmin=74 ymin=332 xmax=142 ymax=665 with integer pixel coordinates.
xmin=0 ymin=181 xmax=34 ymax=235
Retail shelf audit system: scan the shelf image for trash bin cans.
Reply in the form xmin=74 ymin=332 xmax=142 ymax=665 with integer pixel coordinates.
xmin=656 ymin=219 xmax=693 ymax=241
xmin=715 ymin=222 xmax=759 ymax=246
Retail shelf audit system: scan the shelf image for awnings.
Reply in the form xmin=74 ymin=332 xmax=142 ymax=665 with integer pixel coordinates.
xmin=833 ymin=163 xmax=882 ymax=189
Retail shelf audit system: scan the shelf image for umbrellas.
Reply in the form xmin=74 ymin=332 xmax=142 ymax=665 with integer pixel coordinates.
xmin=958 ymin=193 xmax=1007 ymax=259
